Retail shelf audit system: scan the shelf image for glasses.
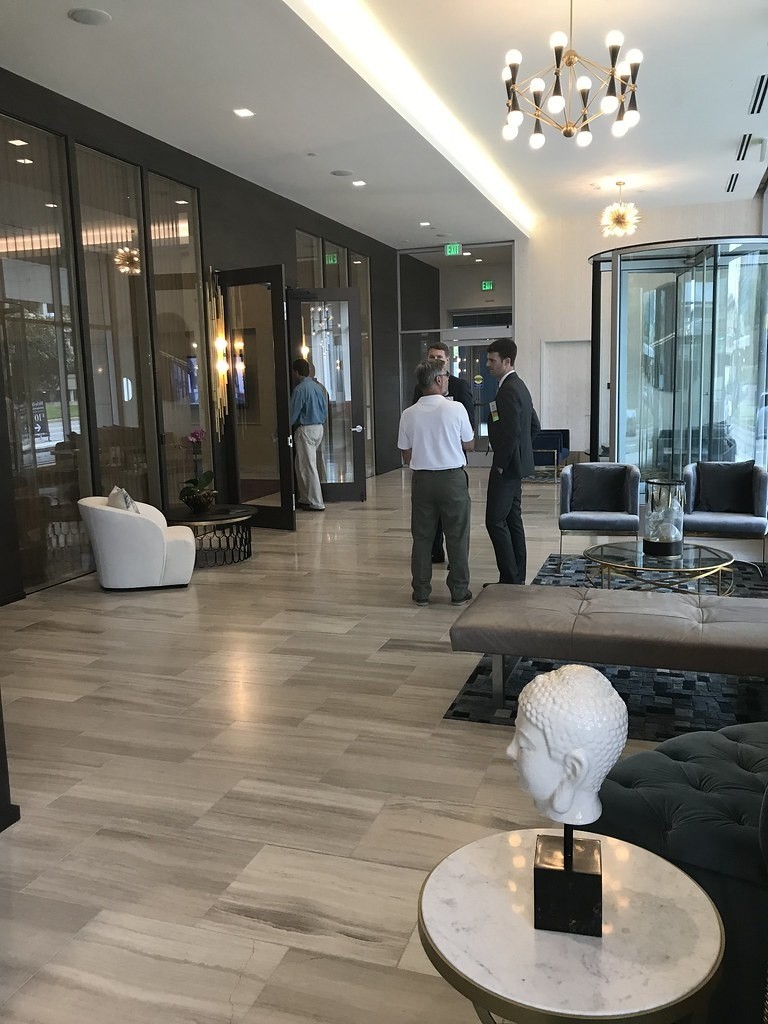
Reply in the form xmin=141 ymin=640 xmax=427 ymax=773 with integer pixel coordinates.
xmin=434 ymin=370 xmax=451 ymax=382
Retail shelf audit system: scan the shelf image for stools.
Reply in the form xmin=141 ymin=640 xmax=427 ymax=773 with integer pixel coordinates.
xmin=580 ymin=722 xmax=768 ymax=1024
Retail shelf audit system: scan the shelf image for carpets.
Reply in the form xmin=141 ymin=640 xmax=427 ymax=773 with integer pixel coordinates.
xmin=521 ymin=468 xmax=562 ymax=483
xmin=444 ymin=553 xmax=768 ymax=743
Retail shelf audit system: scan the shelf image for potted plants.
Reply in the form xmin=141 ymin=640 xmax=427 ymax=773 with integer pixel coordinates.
xmin=179 ymin=471 xmax=217 ymax=510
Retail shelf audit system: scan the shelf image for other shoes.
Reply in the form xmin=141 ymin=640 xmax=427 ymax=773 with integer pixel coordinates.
xmin=450 ymin=590 xmax=472 ymax=605
xmin=300 ymin=505 xmax=326 ymax=511
xmin=484 ymin=581 xmax=501 ymax=587
xmin=412 ymin=592 xmax=429 ymax=606
xmin=295 ymin=501 xmax=311 ymax=508
xmin=447 ymin=564 xmax=451 ymax=570
xmin=431 ymin=552 xmax=446 ymax=563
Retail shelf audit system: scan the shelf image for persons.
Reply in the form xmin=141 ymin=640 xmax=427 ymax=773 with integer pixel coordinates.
xmin=505 ymin=663 xmax=628 ymax=826
xmin=287 ymin=358 xmax=327 ymax=511
xmin=413 ymin=343 xmax=475 ymax=569
xmin=483 ymin=339 xmax=541 ymax=586
xmin=398 ymin=358 xmax=475 ymax=606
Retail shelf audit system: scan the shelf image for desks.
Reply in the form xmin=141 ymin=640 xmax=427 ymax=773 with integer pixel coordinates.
xmin=161 ymin=505 xmax=256 ymax=567
xmin=585 ymin=543 xmax=734 ymax=597
xmin=416 ymin=829 xmax=727 ymax=1024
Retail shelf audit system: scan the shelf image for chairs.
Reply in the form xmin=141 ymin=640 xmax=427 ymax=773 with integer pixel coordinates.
xmin=532 ymin=429 xmax=562 ymax=469
xmin=682 ymin=463 xmax=768 ymax=568
xmin=557 ymin=461 xmax=641 ymax=573
xmin=77 ymin=496 xmax=196 ymax=589
xmin=535 ymin=432 xmax=569 ymax=467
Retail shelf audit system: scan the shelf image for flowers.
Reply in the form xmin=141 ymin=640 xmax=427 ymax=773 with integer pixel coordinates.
xmin=190 ymin=428 xmax=206 ymax=444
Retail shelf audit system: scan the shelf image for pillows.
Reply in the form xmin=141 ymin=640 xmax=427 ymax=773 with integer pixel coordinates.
xmin=570 ymin=464 xmax=627 ymax=511
xmin=694 ymin=459 xmax=756 ymax=509
xmin=108 ymin=485 xmax=138 ymax=514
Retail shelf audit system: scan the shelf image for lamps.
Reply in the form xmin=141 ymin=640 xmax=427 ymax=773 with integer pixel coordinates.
xmin=601 ymin=182 xmax=641 ymax=238
xmin=503 ymin=0 xmax=642 ymax=150
xmin=113 ymin=224 xmax=142 ymax=276
xmin=310 ymin=302 xmax=341 ymax=358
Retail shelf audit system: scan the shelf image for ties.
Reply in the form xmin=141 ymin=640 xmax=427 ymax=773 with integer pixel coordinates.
xmin=495 ymin=384 xmax=500 ymax=397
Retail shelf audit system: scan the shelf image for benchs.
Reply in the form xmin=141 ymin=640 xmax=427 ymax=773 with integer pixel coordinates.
xmin=449 ymin=584 xmax=768 ymax=694
